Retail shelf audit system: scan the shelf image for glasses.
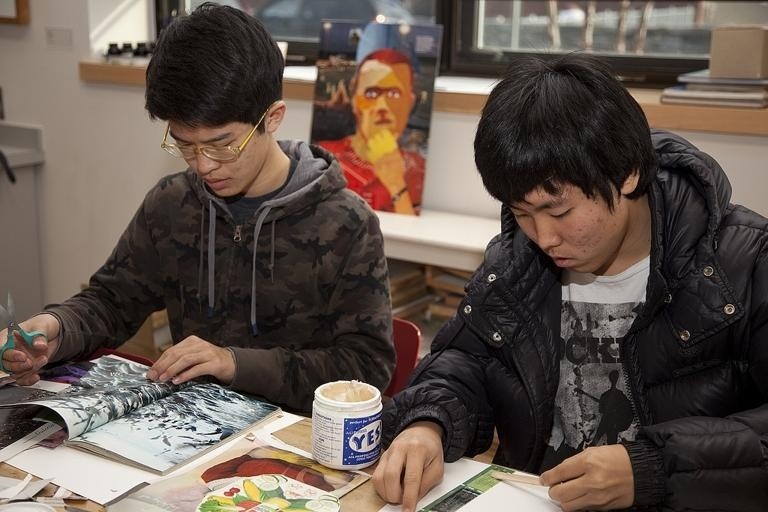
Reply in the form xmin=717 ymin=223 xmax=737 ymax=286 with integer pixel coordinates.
xmin=161 ymin=100 xmax=282 ymax=163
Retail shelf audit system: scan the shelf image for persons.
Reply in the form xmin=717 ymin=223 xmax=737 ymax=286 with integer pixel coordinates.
xmin=0 ymin=2 xmax=398 ymax=415
xmin=311 ymin=48 xmax=425 ymax=216
xmin=372 ymin=50 xmax=766 ymax=510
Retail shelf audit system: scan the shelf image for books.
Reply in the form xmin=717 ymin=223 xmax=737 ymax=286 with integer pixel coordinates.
xmin=3 ymin=353 xmax=283 ymax=478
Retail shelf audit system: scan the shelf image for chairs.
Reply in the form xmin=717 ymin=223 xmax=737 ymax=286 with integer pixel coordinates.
xmin=385 ymin=318 xmax=420 ymax=393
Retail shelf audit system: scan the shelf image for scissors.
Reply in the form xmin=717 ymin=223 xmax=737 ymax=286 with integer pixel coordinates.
xmin=0 ymin=291 xmax=48 ymax=375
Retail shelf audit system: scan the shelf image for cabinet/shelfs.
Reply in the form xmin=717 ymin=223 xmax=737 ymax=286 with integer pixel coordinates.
xmin=0 ymin=118 xmax=46 ymax=329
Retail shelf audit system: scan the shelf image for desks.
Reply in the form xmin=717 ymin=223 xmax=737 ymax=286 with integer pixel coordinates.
xmin=347 ymin=207 xmax=505 ymax=325
xmin=1 ymin=349 xmax=587 ymax=512
